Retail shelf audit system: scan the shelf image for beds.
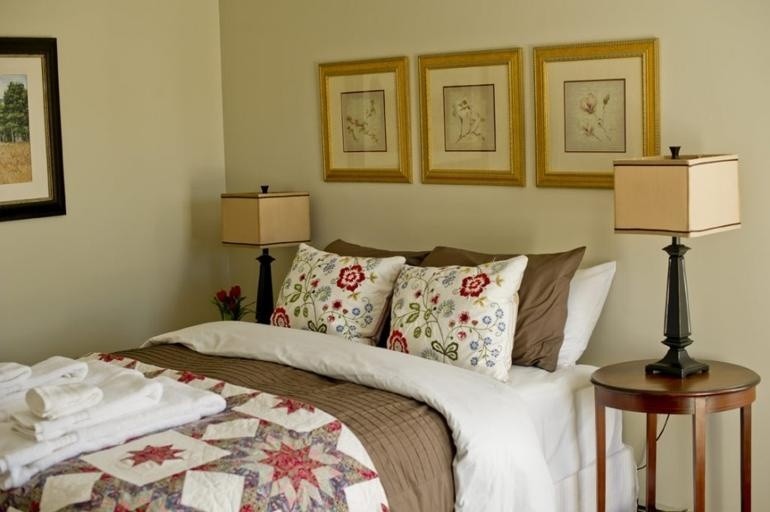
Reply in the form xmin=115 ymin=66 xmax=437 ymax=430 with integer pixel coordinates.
xmin=0 ymin=321 xmax=640 ymax=512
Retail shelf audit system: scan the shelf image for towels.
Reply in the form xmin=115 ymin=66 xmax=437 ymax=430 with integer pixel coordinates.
xmin=0 ymin=355 xmax=227 ymax=493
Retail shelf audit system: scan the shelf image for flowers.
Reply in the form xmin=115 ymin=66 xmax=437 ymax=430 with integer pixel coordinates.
xmin=209 ymin=285 xmax=258 ymax=322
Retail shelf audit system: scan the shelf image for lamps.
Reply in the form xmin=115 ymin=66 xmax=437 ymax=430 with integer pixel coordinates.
xmin=613 ymin=147 xmax=742 ymax=377
xmin=220 ymin=186 xmax=313 ymax=324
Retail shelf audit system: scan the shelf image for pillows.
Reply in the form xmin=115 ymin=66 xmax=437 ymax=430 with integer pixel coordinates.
xmin=549 ymin=258 xmax=615 ymax=372
xmin=271 ymin=239 xmax=404 ymax=354
xmin=419 ymin=244 xmax=587 ymax=376
xmin=388 ymin=255 xmax=529 ymax=382
xmin=323 ymin=241 xmax=427 ymax=276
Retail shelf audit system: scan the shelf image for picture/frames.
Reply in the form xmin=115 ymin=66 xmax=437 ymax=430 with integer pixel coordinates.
xmin=319 ymin=56 xmax=414 ymax=185
xmin=0 ymin=36 xmax=67 ymax=222
xmin=532 ymin=37 xmax=661 ymax=190
xmin=417 ymin=47 xmax=527 ymax=188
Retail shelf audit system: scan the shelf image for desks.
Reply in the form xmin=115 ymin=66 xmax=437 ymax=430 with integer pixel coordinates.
xmin=590 ymin=358 xmax=761 ymax=512
